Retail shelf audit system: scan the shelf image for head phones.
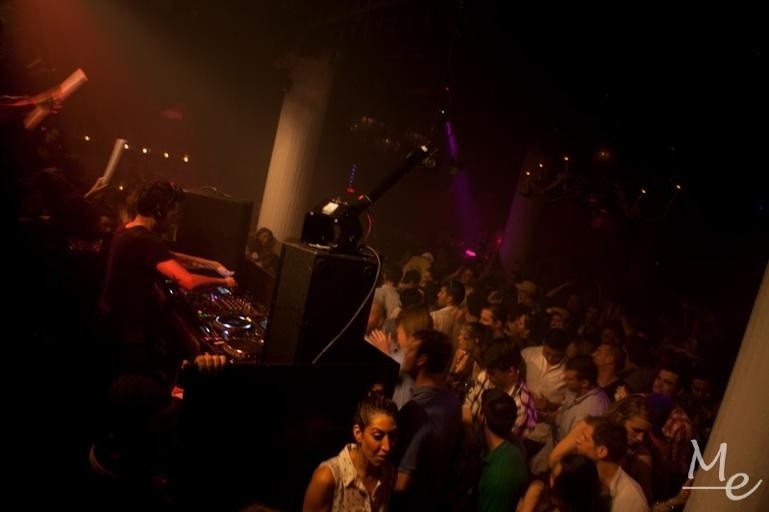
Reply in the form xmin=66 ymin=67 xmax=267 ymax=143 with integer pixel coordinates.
xmin=151 ymin=188 xmax=182 ymax=223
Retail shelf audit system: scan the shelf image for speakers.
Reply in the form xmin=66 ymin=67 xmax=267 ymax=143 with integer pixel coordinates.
xmin=260 ymin=242 xmax=380 ymax=365
xmin=174 ymin=188 xmax=253 ymax=263
